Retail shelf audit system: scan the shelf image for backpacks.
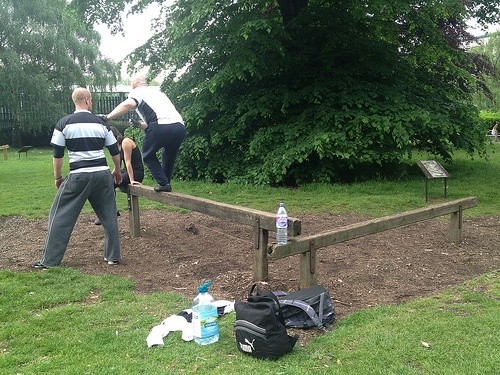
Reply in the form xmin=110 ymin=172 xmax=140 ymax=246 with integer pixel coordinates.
xmin=233 ymin=283 xmax=299 ymax=359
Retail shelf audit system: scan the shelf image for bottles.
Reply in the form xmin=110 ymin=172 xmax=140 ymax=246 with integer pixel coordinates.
xmin=275 ymin=202 xmax=288 ymax=245
xmin=191 ymin=280 xmax=220 ymax=345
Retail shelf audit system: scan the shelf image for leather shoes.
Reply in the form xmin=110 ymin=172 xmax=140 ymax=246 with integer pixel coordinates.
xmin=154 ymin=183 xmax=172 ymax=192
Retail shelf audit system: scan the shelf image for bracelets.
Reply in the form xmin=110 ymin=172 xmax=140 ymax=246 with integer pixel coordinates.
xmin=55 ymin=176 xmax=62 ymax=180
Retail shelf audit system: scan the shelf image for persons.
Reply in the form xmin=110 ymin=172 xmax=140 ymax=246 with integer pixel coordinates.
xmin=96 ymin=75 xmax=185 ymax=191
xmin=33 ymin=87 xmax=122 ymax=268
xmin=94 ymin=126 xmax=144 ymax=225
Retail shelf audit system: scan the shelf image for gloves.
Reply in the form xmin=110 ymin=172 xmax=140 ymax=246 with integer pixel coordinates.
xmin=96 ymin=113 xmax=107 ymax=121
xmin=131 ymin=118 xmax=141 ymax=129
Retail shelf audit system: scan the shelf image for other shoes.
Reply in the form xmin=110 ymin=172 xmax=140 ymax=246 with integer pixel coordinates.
xmin=34 ymin=260 xmax=46 ymax=268
xmin=95 ymin=209 xmax=120 ymax=225
xmin=103 ymin=256 xmax=119 ymax=265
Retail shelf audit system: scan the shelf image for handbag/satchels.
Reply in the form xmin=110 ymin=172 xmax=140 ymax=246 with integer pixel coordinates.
xmin=275 ymin=284 xmax=336 ymax=333
